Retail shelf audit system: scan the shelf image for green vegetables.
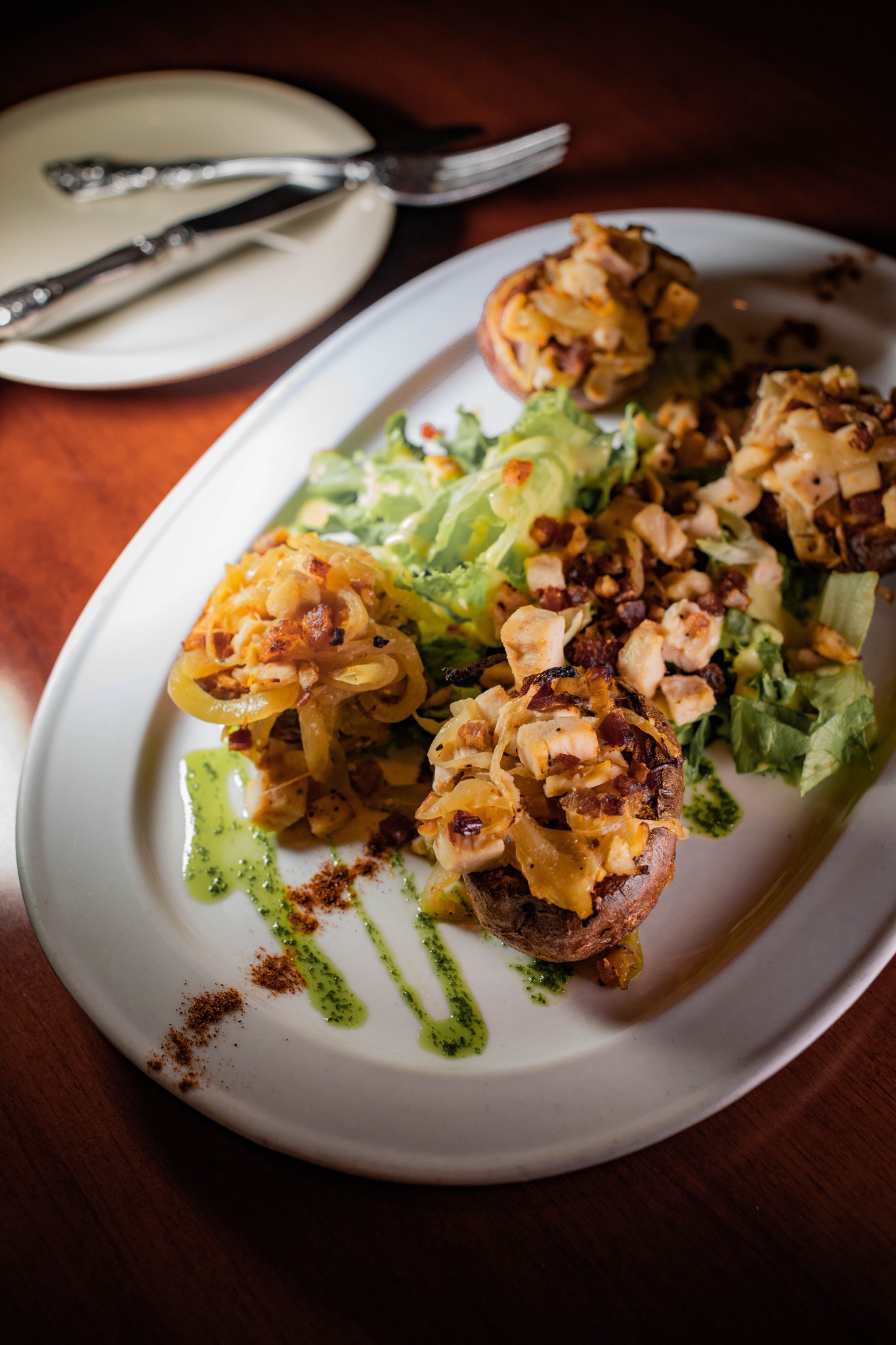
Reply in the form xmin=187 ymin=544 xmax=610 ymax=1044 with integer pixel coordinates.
xmin=296 ymin=389 xmax=892 ymax=793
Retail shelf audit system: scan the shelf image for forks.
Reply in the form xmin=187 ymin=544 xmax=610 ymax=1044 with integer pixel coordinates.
xmin=38 ymin=122 xmax=570 ymax=206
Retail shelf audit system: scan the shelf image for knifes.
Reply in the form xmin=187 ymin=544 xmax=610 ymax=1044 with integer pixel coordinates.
xmin=0 ymin=122 xmax=486 ymax=329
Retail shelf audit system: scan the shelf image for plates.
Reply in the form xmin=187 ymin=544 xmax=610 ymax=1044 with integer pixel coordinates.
xmin=0 ymin=67 xmax=392 ymax=392
xmin=14 ymin=206 xmax=896 ymax=1188
